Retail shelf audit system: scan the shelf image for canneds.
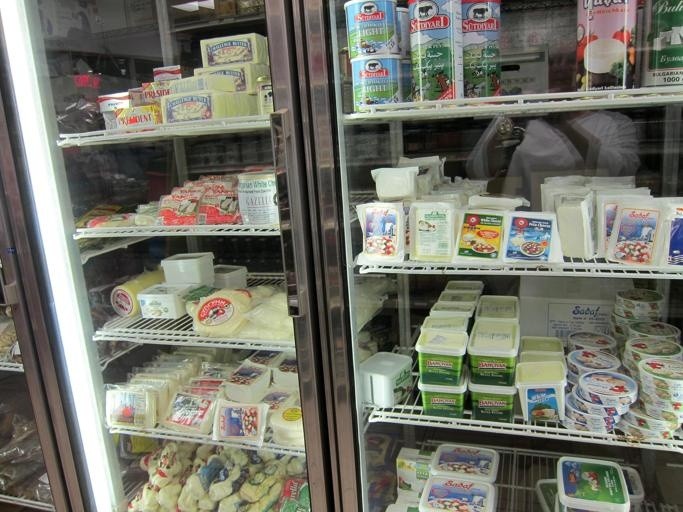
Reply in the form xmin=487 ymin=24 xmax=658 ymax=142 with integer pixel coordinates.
xmin=409 ymin=0 xmax=463 ymax=110
xmin=348 ymin=55 xmax=403 ymax=114
xmin=396 ymin=5 xmax=409 ymax=59
xmin=402 ymin=60 xmax=410 ymax=103
xmin=342 ymin=1 xmax=401 ymax=57
xmin=640 ymin=0 xmax=683 ymax=88
xmin=576 ymin=0 xmax=638 ymax=92
xmin=461 ymin=1 xmax=501 ymax=106
